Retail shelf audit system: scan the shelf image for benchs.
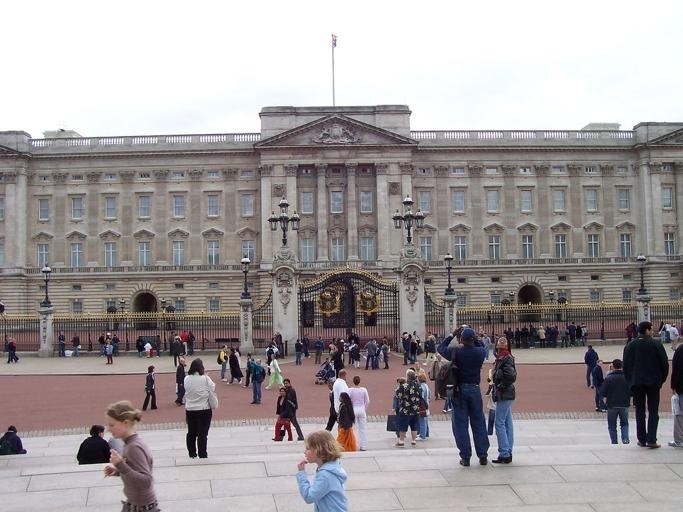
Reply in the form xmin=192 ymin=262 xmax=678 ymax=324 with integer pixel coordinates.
xmin=139 ymin=335 xmax=160 ymax=357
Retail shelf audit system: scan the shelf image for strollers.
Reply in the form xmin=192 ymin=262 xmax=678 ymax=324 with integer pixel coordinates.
xmin=314 ymin=361 xmax=335 ymax=385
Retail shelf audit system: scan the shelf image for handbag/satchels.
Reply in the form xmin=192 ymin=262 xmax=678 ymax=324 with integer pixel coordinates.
xmin=387 ymin=415 xmax=401 ymax=431
xmin=437 ymin=361 xmax=460 ymax=398
xmin=207 ymin=391 xmax=218 ymax=408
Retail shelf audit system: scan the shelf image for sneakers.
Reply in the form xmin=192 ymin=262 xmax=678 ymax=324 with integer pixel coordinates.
xmin=394 ymin=434 xmax=428 ymax=448
xmin=637 ymin=440 xmax=683 ymax=448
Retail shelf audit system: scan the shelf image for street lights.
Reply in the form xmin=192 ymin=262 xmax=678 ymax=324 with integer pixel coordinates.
xmin=39 ymin=261 xmax=51 ymax=307
xmin=267 ymin=195 xmax=300 ymax=246
xmin=239 ymin=255 xmax=250 ymax=299
xmin=635 ymin=253 xmax=646 ymax=295
xmin=443 ymin=253 xmax=455 ymax=295
xmin=391 ymin=194 xmax=425 ymax=243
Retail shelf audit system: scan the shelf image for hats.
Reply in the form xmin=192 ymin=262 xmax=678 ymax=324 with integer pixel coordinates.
xmin=461 ymin=328 xmax=476 ymax=338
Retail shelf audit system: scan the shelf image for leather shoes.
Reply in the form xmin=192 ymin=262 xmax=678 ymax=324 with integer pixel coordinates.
xmin=460 ymin=455 xmax=511 ymax=465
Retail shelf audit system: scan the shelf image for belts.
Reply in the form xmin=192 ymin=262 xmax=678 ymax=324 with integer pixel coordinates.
xmin=121 ymin=500 xmax=156 ymax=512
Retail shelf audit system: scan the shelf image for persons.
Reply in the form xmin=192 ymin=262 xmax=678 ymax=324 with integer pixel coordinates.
xmin=58 ymin=330 xmax=66 ymax=357
xmin=4 ymin=336 xmax=19 ymax=363
xmin=102 ymin=399 xmax=162 ymax=512
xmin=97 ymin=319 xmax=683 ymax=469
xmin=70 ymin=333 xmax=80 ymax=357
xmin=76 ymin=424 xmax=111 ymax=465
xmin=296 ymin=430 xmax=351 ymax=512
xmin=0 ymin=424 xmax=27 ymax=457
